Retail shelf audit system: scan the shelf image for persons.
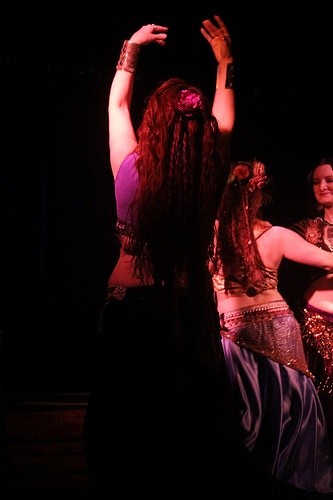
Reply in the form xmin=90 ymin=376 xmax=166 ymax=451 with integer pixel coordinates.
xmin=277 ymin=159 xmax=333 ymax=405
xmin=91 ymin=15 xmax=241 ymax=500
xmin=207 ymin=157 xmax=333 ymax=497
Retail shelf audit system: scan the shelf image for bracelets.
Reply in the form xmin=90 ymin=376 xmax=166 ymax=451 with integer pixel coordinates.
xmin=215 ymin=62 xmax=236 ymax=90
xmin=115 ymin=39 xmax=138 ymax=73
